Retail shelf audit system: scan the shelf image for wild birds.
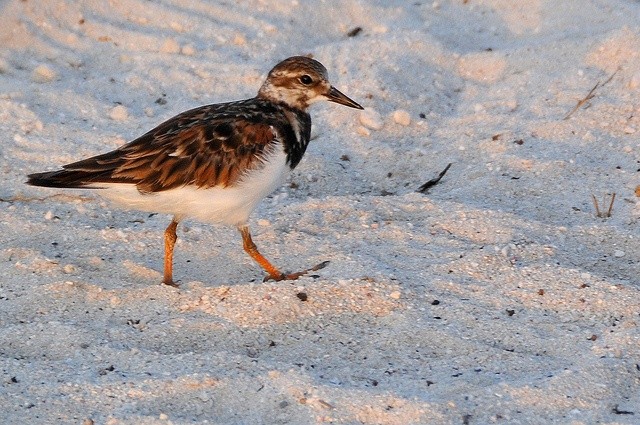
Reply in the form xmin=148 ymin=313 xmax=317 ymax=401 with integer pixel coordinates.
xmin=23 ymin=55 xmax=365 ymax=290
